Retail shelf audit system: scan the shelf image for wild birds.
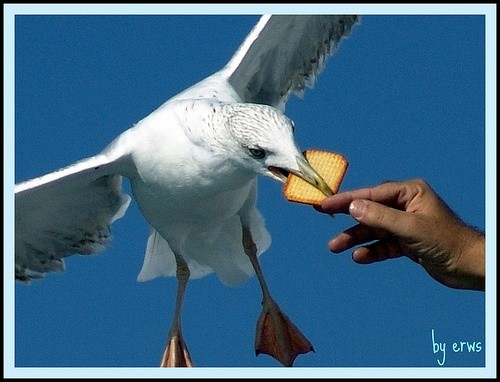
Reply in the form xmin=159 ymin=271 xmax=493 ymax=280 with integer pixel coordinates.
xmin=15 ymin=14 xmax=362 ymax=368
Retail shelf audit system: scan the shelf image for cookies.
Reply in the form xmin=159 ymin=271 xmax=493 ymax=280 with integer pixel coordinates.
xmin=280 ymin=149 xmax=349 ymax=206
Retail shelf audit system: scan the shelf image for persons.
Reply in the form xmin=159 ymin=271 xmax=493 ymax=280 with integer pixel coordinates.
xmin=311 ymin=178 xmax=485 ymax=294
